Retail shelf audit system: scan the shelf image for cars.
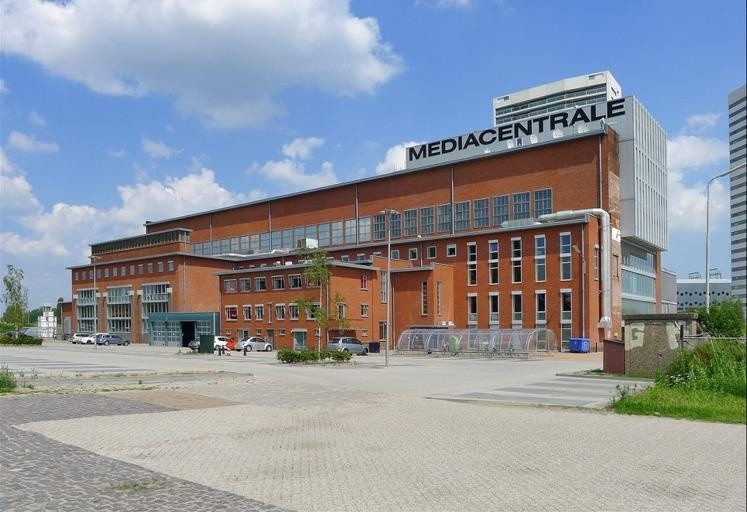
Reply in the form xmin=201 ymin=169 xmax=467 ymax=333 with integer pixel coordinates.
xmin=235 ymin=336 xmax=272 ymax=351
xmin=188 ymin=334 xmax=235 ymax=351
xmin=62 ymin=333 xmax=131 ymax=346
xmin=3 ymin=331 xmax=31 ymax=339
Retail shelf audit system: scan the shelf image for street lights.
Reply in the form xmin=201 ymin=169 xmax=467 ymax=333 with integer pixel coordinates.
xmin=87 ymin=254 xmax=102 ymax=351
xmin=381 ymin=207 xmax=397 ymax=368
xmin=701 ymin=162 xmax=745 ymax=315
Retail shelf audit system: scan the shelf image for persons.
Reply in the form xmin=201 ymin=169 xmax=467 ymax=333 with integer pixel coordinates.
xmin=441 ymin=339 xmax=514 ymax=358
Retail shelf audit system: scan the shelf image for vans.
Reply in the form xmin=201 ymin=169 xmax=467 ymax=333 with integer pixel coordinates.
xmin=327 ymin=335 xmax=369 ymax=355
